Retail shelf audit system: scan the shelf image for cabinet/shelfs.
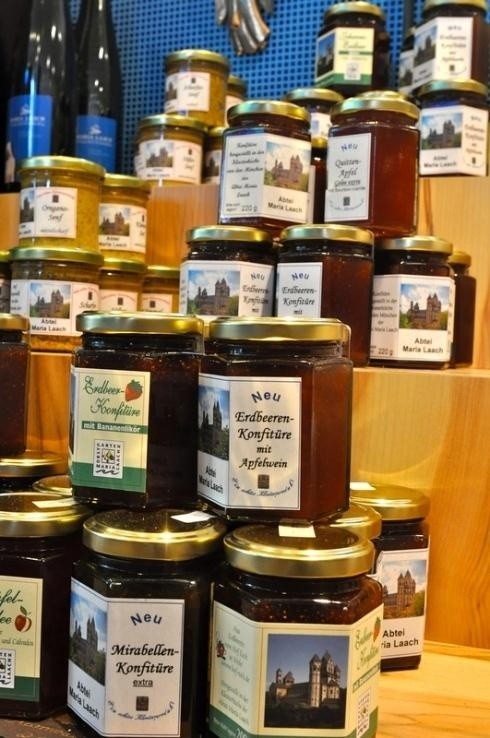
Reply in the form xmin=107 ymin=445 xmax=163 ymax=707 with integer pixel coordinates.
xmin=0 ymin=174 xmax=490 ymax=738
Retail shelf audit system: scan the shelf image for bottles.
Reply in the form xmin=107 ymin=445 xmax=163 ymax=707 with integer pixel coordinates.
xmin=67 ymin=0 xmax=123 ymax=173
xmin=0 ymin=0 xmax=77 ymax=192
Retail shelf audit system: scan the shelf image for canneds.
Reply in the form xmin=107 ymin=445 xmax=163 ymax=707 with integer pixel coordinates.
xmin=216 ymin=99 xmax=314 ymax=235
xmin=194 ymin=313 xmax=356 ymax=526
xmin=64 ymin=502 xmax=222 ymax=738
xmin=308 ymin=133 xmax=328 ymax=225
xmin=0 ymin=492 xmax=95 ymax=724
xmin=99 ymin=259 xmax=149 ymax=315
xmin=224 ymin=75 xmax=248 ymax=132
xmin=369 ymin=234 xmax=460 ymax=371
xmin=14 ymin=154 xmax=107 ymax=256
xmin=396 ymin=27 xmax=418 ymax=96
xmin=66 ymin=310 xmax=201 ymax=515
xmin=0 ymin=250 xmax=15 ymax=315
xmin=199 ymin=125 xmax=227 ymax=186
xmin=347 ymin=479 xmax=432 ymax=673
xmin=268 ymin=222 xmax=378 ymax=369
xmin=161 ymin=48 xmax=232 ymax=129
xmin=284 ymin=88 xmax=346 ymax=138
xmin=137 ymin=262 xmax=182 ymax=315
xmin=0 ymin=310 xmax=40 ymax=459
xmin=412 ymin=78 xmax=489 ymax=178
xmin=30 ymin=468 xmax=76 ymax=497
xmin=132 ymin=114 xmax=207 ymax=184
xmin=8 ymin=244 xmax=104 ymax=354
xmin=413 ymin=0 xmax=489 ymax=103
xmin=0 ymin=448 xmax=51 ymax=489
xmin=446 ymin=249 xmax=477 ymax=370
xmin=328 ymin=501 xmax=382 ymax=578
xmin=313 ymin=0 xmax=391 ymax=94
xmin=96 ymin=171 xmax=154 ymax=266
xmin=206 ymin=517 xmax=385 ymax=738
xmin=320 ymin=96 xmax=422 ymax=240
xmin=176 ymin=221 xmax=276 ymax=319
